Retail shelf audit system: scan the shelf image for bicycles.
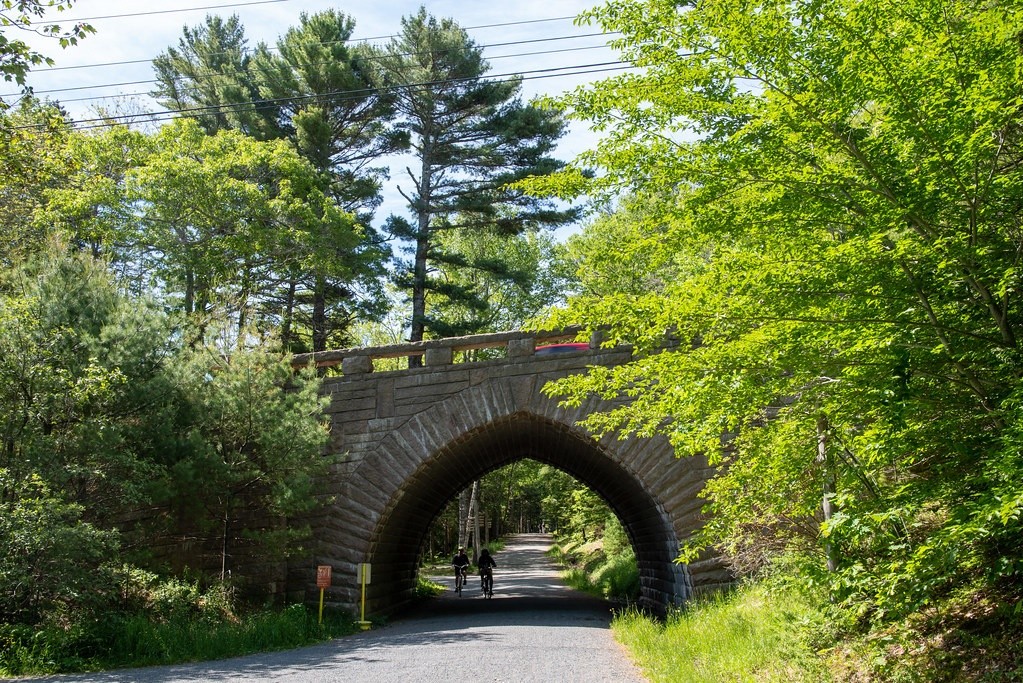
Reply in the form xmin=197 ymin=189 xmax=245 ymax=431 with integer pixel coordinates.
xmin=453 ymin=564 xmax=467 ymax=597
xmin=478 ymin=565 xmax=496 ymax=600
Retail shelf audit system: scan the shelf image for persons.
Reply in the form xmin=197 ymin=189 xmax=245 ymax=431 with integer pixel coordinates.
xmin=452 ymin=547 xmax=470 ymax=592
xmin=479 ymin=549 xmax=497 ymax=595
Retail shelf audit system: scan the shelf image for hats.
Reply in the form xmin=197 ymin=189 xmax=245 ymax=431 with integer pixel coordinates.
xmin=458 ymin=547 xmax=464 ymax=551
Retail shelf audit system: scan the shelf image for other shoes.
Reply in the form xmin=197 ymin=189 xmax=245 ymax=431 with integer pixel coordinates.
xmin=489 ymin=590 xmax=493 ymax=595
xmin=455 ymin=587 xmax=458 ymax=593
xmin=463 ymin=580 xmax=466 ymax=585
